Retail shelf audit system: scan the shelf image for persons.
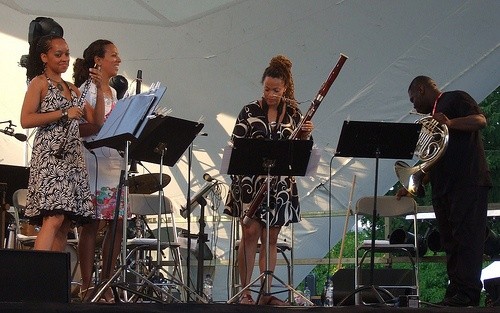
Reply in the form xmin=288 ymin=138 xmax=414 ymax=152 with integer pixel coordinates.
xmin=21 ymin=34 xmax=134 ymax=304
xmin=396 ymin=76 xmax=494 ymax=307
xmin=223 ymin=54 xmax=314 ymax=305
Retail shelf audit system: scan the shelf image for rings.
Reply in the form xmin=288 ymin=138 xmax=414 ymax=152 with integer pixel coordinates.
xmin=308 ymin=129 xmax=311 ymax=131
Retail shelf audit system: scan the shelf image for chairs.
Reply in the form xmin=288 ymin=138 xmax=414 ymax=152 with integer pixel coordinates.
xmin=13 ymin=189 xmax=79 ymax=251
xmin=232 ymin=224 xmax=293 ymax=304
xmin=355 ymin=196 xmax=417 ymax=305
xmin=128 ymin=194 xmax=185 ymax=302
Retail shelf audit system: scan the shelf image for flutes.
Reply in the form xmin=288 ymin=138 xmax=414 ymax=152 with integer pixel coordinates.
xmin=54 ymin=63 xmax=98 ymax=159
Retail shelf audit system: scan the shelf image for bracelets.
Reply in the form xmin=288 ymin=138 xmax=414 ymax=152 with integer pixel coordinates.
xmin=61 ymin=109 xmax=68 ymax=121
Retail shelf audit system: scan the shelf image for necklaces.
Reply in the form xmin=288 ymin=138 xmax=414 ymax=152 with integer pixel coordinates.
xmin=49 ymin=77 xmax=64 ymax=91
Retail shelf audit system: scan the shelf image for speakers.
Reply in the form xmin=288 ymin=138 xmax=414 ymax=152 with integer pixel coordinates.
xmin=0 ymin=248 xmax=71 ymax=303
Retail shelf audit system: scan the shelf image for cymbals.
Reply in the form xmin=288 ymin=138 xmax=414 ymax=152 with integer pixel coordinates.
xmin=128 ymin=172 xmax=171 ymax=195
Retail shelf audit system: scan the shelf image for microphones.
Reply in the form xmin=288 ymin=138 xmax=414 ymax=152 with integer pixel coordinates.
xmin=0 ymin=129 xmax=27 ymax=142
xmin=83 ymin=140 xmax=95 ymax=155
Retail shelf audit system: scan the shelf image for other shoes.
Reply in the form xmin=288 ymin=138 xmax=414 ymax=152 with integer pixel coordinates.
xmin=268 ymin=296 xmax=290 ymax=306
xmin=240 ymin=295 xmax=256 ymax=305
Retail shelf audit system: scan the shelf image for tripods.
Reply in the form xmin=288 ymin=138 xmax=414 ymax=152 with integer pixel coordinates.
xmin=338 ymin=120 xmax=422 ymax=306
xmin=225 ymin=137 xmax=314 ymax=306
xmin=88 ymin=87 xmax=208 ymax=303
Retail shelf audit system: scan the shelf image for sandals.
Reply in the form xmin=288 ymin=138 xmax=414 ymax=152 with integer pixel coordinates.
xmin=99 ymin=280 xmax=115 ymax=303
xmin=80 ymin=287 xmax=106 ymax=303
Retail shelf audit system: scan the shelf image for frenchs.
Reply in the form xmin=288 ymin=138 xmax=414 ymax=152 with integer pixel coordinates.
xmin=394 ymin=110 xmax=449 ymax=197
